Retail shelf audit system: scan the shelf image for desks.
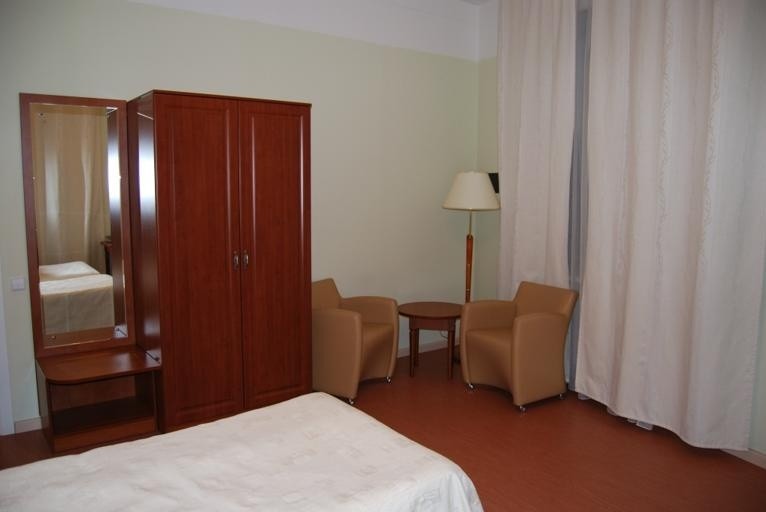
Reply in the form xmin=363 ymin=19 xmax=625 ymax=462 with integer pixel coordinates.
xmin=397 ymin=301 xmax=464 ymax=377
xmin=100 ymin=240 xmax=112 ymax=275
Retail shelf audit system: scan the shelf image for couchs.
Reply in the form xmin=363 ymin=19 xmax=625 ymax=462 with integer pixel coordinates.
xmin=312 ymin=278 xmax=400 ymax=405
xmin=460 ymin=281 xmax=580 ymax=411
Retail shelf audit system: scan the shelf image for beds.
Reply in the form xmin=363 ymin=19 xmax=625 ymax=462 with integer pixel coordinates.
xmin=1 ymin=393 xmax=485 ymax=511
xmin=40 ymin=274 xmax=114 ymax=335
xmin=39 ymin=259 xmax=100 ymax=279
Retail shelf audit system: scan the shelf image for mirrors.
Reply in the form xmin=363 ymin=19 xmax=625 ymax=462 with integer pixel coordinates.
xmin=18 ymin=91 xmax=138 ymax=359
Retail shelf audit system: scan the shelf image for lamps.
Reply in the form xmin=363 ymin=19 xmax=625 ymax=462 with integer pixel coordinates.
xmin=441 ymin=171 xmax=501 ymax=303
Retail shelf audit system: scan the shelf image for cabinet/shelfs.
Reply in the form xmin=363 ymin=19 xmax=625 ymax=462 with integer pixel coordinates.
xmin=127 ymin=89 xmax=314 ymax=433
xmin=34 ymin=358 xmax=160 ymax=456
